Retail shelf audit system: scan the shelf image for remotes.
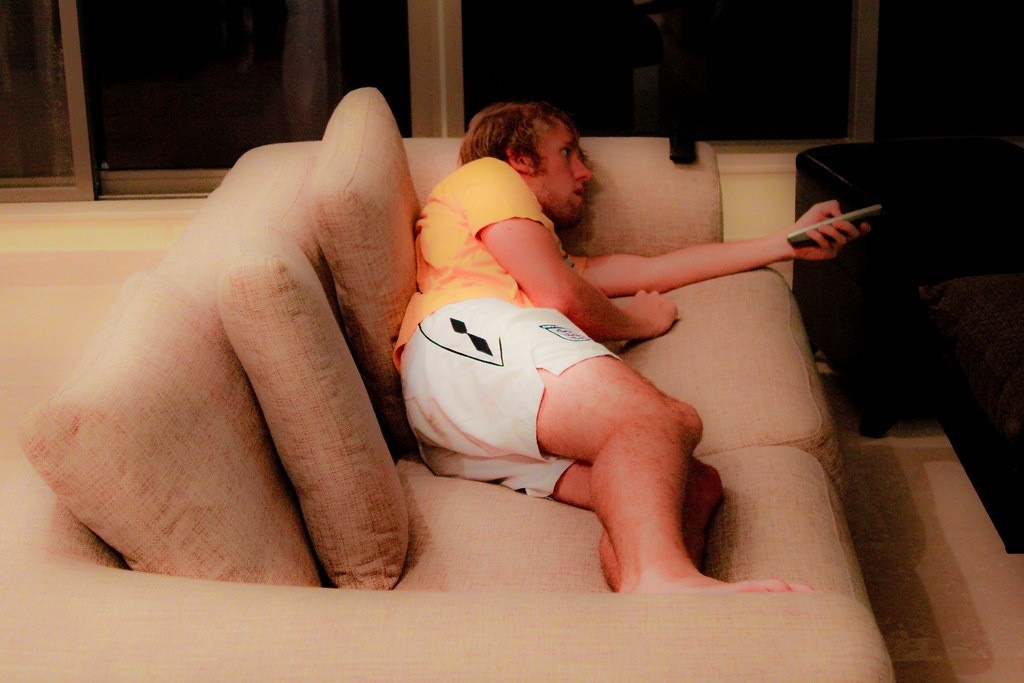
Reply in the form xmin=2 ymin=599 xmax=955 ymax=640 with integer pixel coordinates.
xmin=787 ymin=203 xmax=885 ymax=247
xmin=668 ymin=125 xmax=696 ymax=164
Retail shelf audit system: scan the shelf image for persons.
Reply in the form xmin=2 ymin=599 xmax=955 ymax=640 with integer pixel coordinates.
xmin=392 ymin=100 xmax=872 ymax=594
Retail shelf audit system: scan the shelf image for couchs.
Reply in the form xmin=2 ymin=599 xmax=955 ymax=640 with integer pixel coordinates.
xmin=0 ymin=136 xmax=894 ymax=683
xmin=792 ymin=136 xmax=1023 ymax=438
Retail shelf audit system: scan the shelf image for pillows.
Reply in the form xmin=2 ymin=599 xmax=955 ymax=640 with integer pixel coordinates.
xmin=299 ymin=86 xmax=421 ymax=449
xmin=215 ymin=217 xmax=410 ymax=592
xmin=915 ymin=271 xmax=1024 ymax=447
